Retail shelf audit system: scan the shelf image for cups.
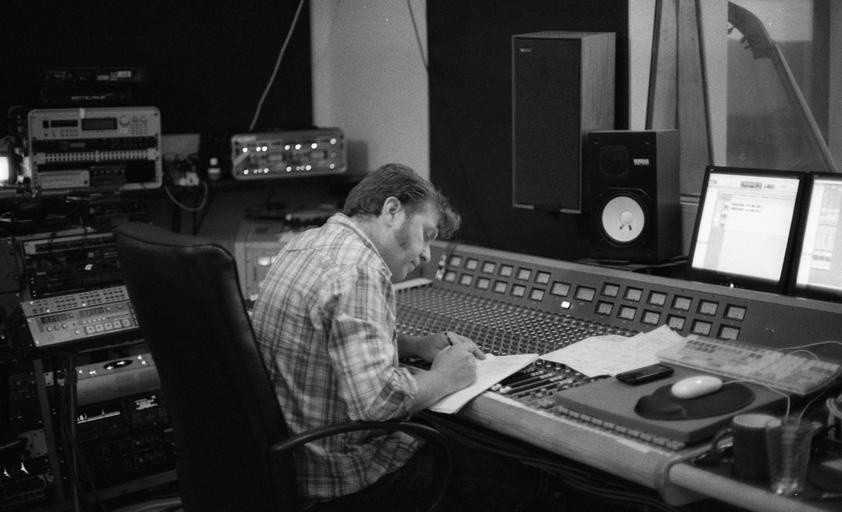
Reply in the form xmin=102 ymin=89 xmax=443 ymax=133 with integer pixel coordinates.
xmin=712 ymin=413 xmax=781 ymax=487
xmin=764 ymin=417 xmax=814 ymax=496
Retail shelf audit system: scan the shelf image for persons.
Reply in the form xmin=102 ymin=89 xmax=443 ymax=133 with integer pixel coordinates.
xmin=249 ymin=162 xmax=564 ymax=510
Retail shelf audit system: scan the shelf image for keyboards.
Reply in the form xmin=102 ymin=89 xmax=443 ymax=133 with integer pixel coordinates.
xmin=666 ymin=329 xmax=835 ymax=403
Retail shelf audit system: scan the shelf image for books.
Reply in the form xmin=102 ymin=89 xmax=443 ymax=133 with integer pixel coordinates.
xmin=423 ymin=350 xmax=540 ymax=416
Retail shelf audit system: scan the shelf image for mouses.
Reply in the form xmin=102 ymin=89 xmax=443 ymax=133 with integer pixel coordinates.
xmin=671 ymin=374 xmax=725 ymax=400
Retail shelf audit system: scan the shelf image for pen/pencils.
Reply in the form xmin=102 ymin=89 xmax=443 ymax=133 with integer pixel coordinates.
xmin=444 ymin=332 xmax=453 ymax=346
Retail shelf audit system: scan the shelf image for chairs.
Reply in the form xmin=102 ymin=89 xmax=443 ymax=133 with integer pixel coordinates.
xmin=114 ymin=223 xmax=459 ymax=512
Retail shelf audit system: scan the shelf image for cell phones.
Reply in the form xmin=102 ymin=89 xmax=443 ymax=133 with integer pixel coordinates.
xmin=614 ymin=361 xmax=674 ymax=384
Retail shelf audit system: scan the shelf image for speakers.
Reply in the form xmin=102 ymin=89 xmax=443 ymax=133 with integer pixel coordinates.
xmin=587 ymin=128 xmax=684 ymax=268
xmin=510 ymin=31 xmax=617 ymax=216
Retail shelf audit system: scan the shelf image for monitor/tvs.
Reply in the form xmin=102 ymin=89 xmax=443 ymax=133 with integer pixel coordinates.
xmin=786 ymin=170 xmax=842 ymax=304
xmin=686 ymin=165 xmax=807 ymax=294
